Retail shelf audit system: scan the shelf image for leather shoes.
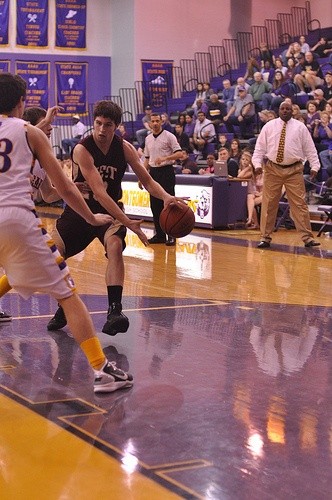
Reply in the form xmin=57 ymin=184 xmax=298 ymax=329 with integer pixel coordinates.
xmin=257 ymin=241 xmax=270 ymax=248
xmin=305 ymin=241 xmax=321 ymax=247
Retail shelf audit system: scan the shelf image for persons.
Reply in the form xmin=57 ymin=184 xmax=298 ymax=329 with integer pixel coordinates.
xmin=252 ymin=102 xmax=321 ymax=247
xmin=136 ymin=106 xmax=155 ymax=146
xmin=176 ymin=83 xmax=228 ymax=161
xmin=259 ymin=71 xmax=292 ymax=109
xmin=47 ymin=100 xmax=190 ymax=336
xmin=198 ymin=134 xmax=264 ymax=230
xmin=119 ymin=124 xmax=134 ymax=145
xmin=243 ymin=36 xmax=332 ymax=96
xmin=0 ymin=105 xmax=92 ymax=321
xmin=220 ymin=72 xmax=273 ymax=141
xmin=160 ymin=113 xmax=176 ymax=134
xmin=126 ymin=144 xmax=198 ymax=174
xmin=0 ymin=73 xmax=134 ymax=395
xmin=259 ymin=100 xmax=332 ymax=184
xmin=55 ymin=156 xmax=73 ymax=182
xmin=62 ymin=115 xmax=88 ymax=156
xmin=136 ymin=114 xmax=184 ymax=245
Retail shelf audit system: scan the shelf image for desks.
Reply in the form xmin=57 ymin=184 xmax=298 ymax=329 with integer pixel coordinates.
xmin=121 ymin=172 xmax=248 ymax=229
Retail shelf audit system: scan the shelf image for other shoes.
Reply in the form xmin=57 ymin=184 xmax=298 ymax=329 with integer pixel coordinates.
xmin=166 ymin=237 xmax=176 ymax=245
xmin=0 ymin=310 xmax=12 ymax=322
xmin=147 ymin=233 xmax=166 ymax=243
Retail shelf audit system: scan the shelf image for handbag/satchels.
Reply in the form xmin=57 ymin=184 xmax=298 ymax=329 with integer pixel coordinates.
xmin=37 ymin=145 xmax=63 ymax=204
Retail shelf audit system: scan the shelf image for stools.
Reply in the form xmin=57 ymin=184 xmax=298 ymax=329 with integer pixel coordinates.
xmin=274 ymin=201 xmax=288 ymax=231
xmin=316 ymin=206 xmax=332 ymax=237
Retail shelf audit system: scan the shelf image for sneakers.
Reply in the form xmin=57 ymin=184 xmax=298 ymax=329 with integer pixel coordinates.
xmin=102 ymin=303 xmax=130 ymax=336
xmin=93 ymin=359 xmax=134 ymax=393
xmin=46 ymin=307 xmax=67 ymax=330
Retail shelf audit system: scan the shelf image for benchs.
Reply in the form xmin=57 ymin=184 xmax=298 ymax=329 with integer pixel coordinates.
xmin=118 ymin=27 xmax=332 ymax=159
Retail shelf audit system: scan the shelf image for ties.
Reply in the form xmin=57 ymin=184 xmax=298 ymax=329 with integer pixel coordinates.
xmin=276 ymin=122 xmax=287 ymax=163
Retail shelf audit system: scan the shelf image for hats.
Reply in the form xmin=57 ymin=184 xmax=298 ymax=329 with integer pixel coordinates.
xmin=237 ymin=86 xmax=245 ymax=91
xmin=145 ymin=106 xmax=152 ymax=110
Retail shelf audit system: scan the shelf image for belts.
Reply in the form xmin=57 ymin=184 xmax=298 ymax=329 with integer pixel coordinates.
xmin=272 ymin=161 xmax=300 ymax=168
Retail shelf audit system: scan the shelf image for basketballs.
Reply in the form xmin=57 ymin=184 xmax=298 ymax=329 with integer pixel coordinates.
xmin=158 ymin=203 xmax=195 ymax=238
